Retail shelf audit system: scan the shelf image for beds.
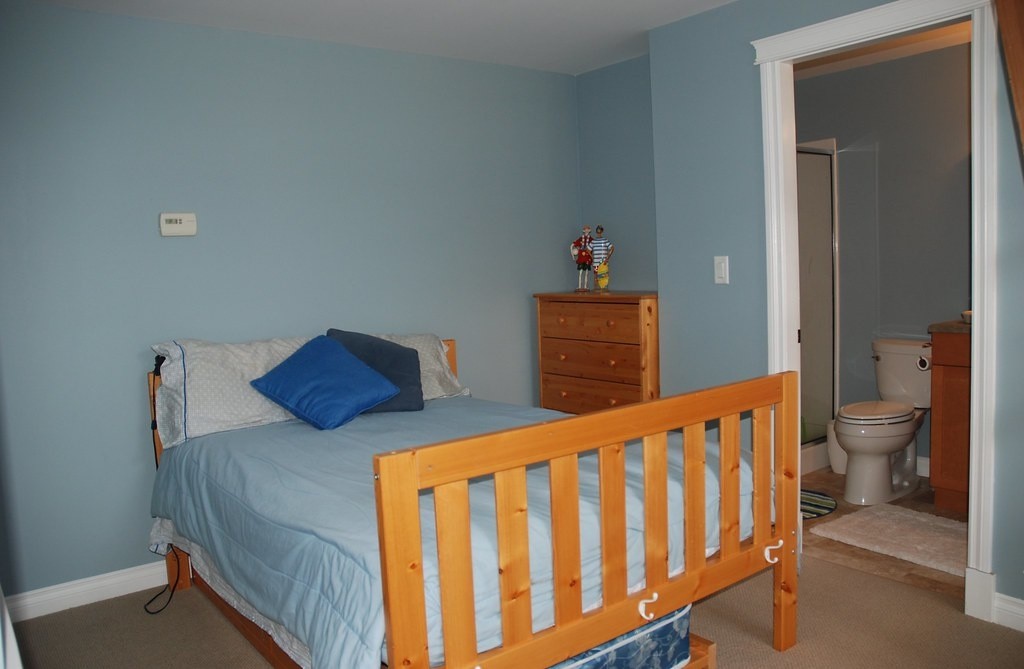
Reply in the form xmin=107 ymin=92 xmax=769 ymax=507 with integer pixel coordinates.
xmin=147 ymin=340 xmax=799 ymax=668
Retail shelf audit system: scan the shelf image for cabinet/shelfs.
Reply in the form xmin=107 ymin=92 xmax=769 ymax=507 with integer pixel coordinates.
xmin=928 ymin=319 xmax=969 ymax=508
xmin=534 ymin=289 xmax=660 ymax=415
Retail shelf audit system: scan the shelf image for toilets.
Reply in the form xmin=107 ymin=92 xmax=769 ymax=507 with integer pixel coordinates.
xmin=832 ymin=336 xmax=936 ymax=507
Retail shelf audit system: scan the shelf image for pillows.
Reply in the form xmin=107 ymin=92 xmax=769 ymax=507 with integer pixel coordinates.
xmin=379 ymin=334 xmax=471 ymax=400
xmin=326 ymin=328 xmax=424 ymax=414
xmin=251 ymin=335 xmax=401 ymax=428
xmin=151 ymin=338 xmax=311 ymax=449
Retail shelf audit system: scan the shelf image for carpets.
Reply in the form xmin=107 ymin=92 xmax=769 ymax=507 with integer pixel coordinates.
xmin=810 ymin=503 xmax=967 ymax=580
xmin=800 ymin=488 xmax=836 ymax=521
